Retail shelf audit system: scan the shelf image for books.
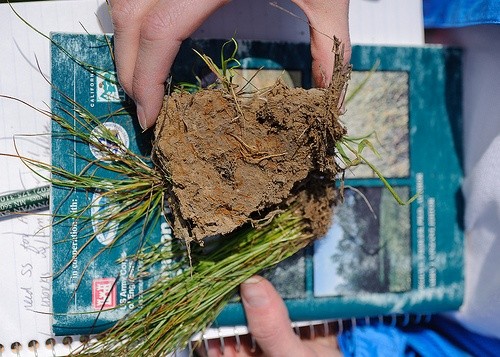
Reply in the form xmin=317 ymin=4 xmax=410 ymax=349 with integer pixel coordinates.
xmin=48 ymin=32 xmax=467 ymax=346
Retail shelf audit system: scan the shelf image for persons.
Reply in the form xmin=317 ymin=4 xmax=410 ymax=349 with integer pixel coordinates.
xmin=106 ymin=0 xmax=499 ymax=356
